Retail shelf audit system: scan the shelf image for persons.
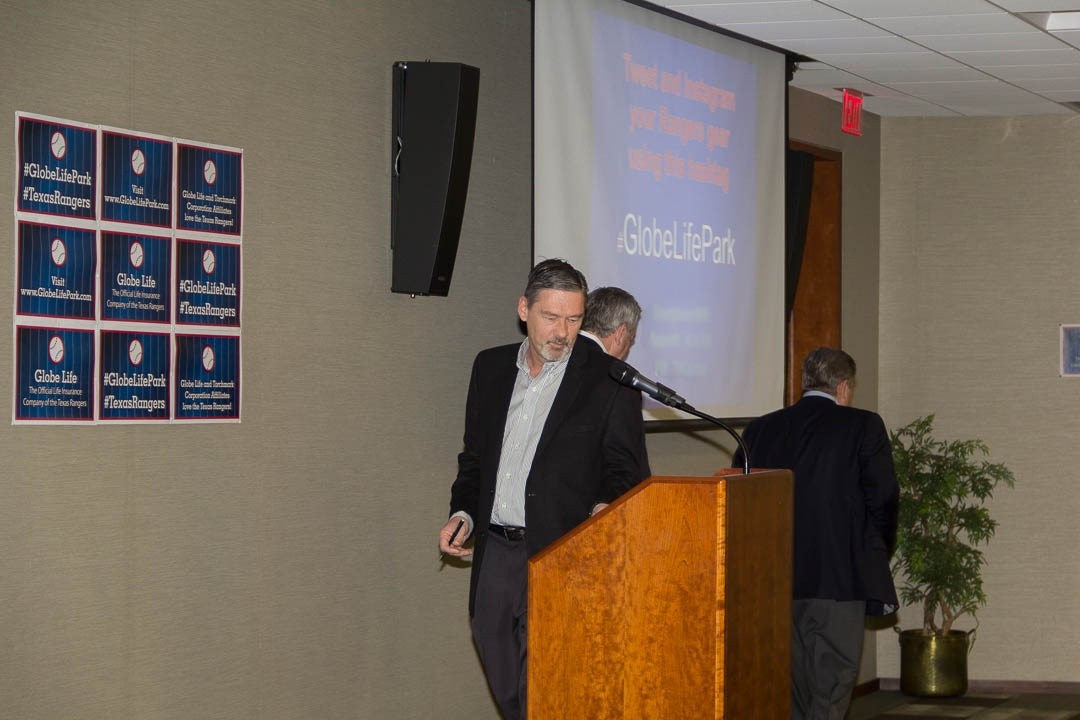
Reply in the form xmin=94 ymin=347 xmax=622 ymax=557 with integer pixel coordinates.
xmin=439 ymin=258 xmax=651 ymax=720
xmin=729 ymin=343 xmax=903 ymax=720
xmin=573 ymin=285 xmax=642 ymax=360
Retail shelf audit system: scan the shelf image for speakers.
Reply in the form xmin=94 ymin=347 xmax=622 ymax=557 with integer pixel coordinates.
xmin=390 ymin=60 xmax=482 ymax=298
xmin=784 ymin=149 xmax=816 ymax=317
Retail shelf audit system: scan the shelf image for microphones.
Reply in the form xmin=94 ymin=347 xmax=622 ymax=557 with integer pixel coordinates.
xmin=607 ymin=359 xmax=695 ymax=414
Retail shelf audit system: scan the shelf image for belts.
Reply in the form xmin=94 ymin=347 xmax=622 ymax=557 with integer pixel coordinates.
xmin=489 ymin=525 xmax=528 ymax=542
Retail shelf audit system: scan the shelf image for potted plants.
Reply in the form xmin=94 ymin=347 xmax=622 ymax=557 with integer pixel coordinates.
xmin=889 ymin=414 xmax=1016 ymax=699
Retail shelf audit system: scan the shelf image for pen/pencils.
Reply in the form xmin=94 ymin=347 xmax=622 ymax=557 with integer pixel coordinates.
xmin=439 ymin=519 xmax=464 ymax=562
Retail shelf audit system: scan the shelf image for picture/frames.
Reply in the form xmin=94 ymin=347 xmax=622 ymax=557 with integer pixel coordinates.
xmin=1059 ymin=323 xmax=1080 ymax=378
